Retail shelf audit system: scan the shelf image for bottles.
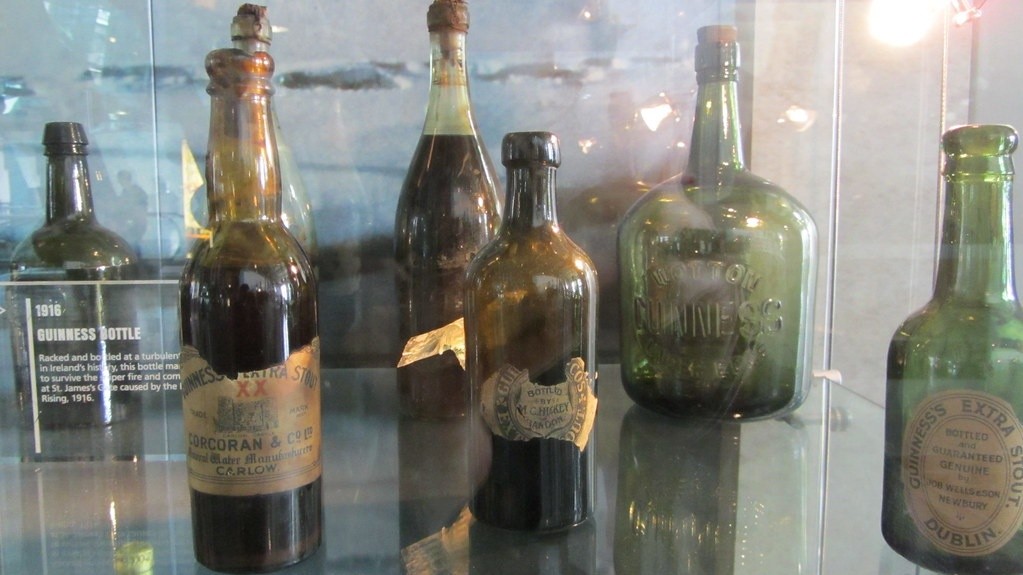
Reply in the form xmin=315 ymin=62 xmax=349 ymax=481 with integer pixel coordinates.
xmin=617 ymin=22 xmax=820 ymax=416
xmin=180 ymin=50 xmax=326 ymax=570
xmin=459 ymin=129 xmax=599 ymax=532
xmin=881 ymin=125 xmax=1023 ymax=575
xmin=7 ymin=123 xmax=144 ymax=429
xmin=221 ymin=2 xmax=318 ymax=252
xmin=391 ymin=1 xmax=500 ymax=416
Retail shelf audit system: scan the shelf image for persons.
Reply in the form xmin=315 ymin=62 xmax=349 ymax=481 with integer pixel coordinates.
xmin=118 ymin=171 xmax=148 ymax=261
